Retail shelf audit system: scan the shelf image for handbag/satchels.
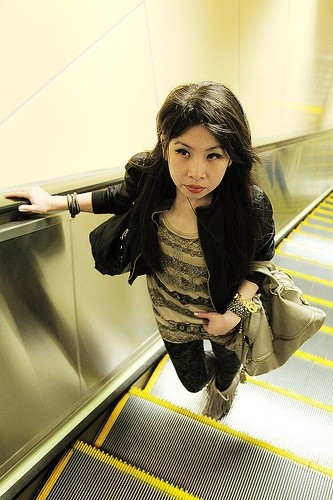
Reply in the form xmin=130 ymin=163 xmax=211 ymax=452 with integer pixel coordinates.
xmin=89 ymin=204 xmax=140 ymax=276
xmin=225 ymin=260 xmax=328 ymax=383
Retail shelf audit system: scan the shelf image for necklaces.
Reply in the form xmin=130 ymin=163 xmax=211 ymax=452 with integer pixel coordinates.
xmin=187 ymin=197 xmax=226 ymax=251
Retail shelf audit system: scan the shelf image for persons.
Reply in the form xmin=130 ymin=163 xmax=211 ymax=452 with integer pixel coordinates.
xmin=3 ymin=81 xmax=275 ymax=421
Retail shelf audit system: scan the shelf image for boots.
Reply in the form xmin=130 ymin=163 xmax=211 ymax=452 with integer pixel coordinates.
xmin=201 ymin=370 xmax=241 ymax=422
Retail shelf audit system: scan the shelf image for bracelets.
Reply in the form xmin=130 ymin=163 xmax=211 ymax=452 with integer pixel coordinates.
xmin=228 ymin=292 xmax=257 ymax=321
xmin=67 ymin=192 xmax=81 ymax=219
